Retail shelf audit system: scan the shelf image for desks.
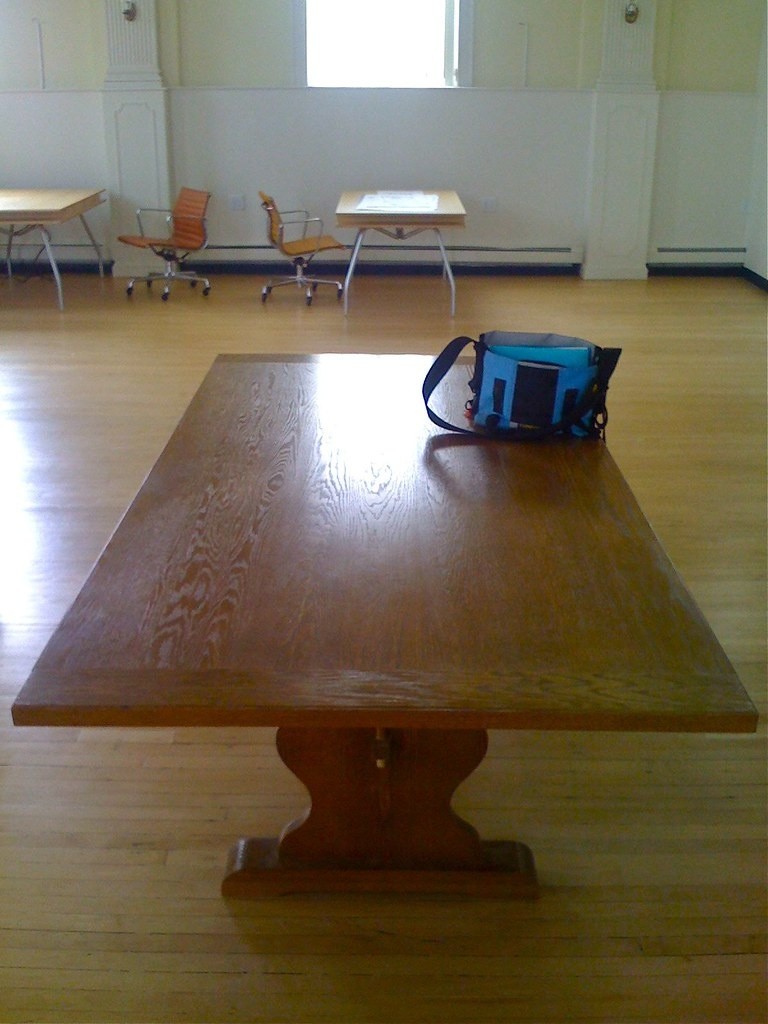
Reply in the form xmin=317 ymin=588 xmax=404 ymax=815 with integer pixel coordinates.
xmin=334 ymin=190 xmax=468 ymax=319
xmin=10 ymin=352 xmax=761 ymax=902
xmin=0 ymin=188 xmax=108 ymax=311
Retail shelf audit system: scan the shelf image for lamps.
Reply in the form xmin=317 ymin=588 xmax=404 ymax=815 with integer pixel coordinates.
xmin=121 ymin=0 xmax=136 ymax=21
xmin=625 ymin=0 xmax=639 ymax=24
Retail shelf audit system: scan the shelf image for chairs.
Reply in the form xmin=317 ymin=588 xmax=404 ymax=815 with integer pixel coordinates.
xmin=117 ymin=187 xmax=213 ymax=301
xmin=258 ymin=191 xmax=346 ymax=307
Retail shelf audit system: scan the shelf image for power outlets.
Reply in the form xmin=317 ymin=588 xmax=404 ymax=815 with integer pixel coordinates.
xmin=230 ymin=194 xmax=245 ymax=212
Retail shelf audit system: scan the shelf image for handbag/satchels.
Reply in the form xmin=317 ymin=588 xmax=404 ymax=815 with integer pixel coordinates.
xmin=422 ymin=331 xmax=622 ymax=440
